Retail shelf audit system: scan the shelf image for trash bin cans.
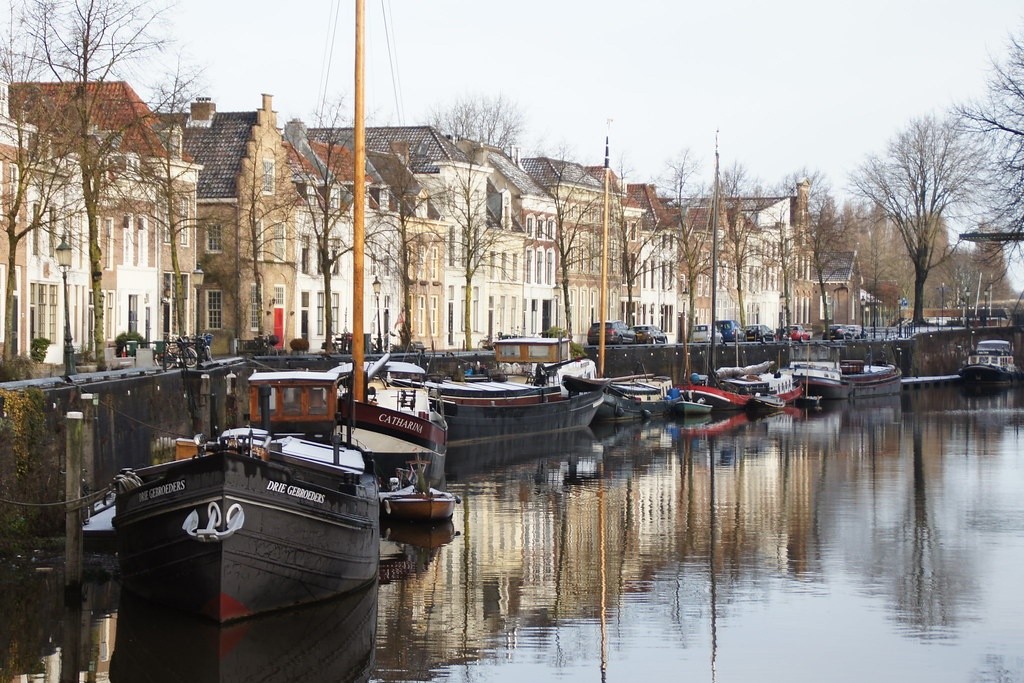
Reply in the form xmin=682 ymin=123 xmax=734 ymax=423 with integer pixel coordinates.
xmin=153 ymin=341 xmax=166 ymax=359
xmin=126 ymin=341 xmax=138 ymax=355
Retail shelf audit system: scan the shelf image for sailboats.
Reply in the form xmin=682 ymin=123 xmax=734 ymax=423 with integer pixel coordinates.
xmin=669 ymin=151 xmax=804 ymax=411
xmin=674 ymin=301 xmax=713 ymax=415
xmin=560 ymin=135 xmax=684 ymax=420
xmin=279 ymin=0 xmax=449 ymax=485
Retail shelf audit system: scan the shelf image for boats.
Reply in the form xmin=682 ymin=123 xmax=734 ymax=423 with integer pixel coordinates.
xmin=958 ymin=341 xmax=1023 ymax=386
xmin=382 ymin=520 xmax=453 ymax=549
xmin=387 ymin=338 xmax=606 ymax=451
xmin=789 ymin=345 xmax=903 ymax=397
xmin=109 ymin=585 xmax=378 ymax=683
xmin=379 ymin=481 xmax=456 ymax=521
xmin=111 ymin=370 xmax=381 ymax=625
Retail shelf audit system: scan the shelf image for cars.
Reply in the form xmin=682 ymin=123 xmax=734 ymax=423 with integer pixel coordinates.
xmin=588 ymin=320 xmax=861 ymax=343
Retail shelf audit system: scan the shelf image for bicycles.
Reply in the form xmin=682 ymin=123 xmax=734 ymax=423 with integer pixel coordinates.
xmin=154 ymin=330 xmax=212 ymax=369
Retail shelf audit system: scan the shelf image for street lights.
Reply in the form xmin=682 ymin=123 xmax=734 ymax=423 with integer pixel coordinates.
xmin=56 ymin=235 xmax=76 ymax=376
xmin=861 ymin=296 xmax=865 ymax=339
xmin=192 ymin=262 xmax=204 ymax=368
xmin=939 ymin=282 xmax=989 ymax=328
xmin=372 ymin=276 xmax=382 ymax=353
xmin=683 ymin=287 xmax=689 ymax=311
xmin=780 ymin=291 xmax=786 ymax=343
xmin=897 ymin=295 xmax=902 ymax=338
xmin=553 ymin=281 xmax=560 ymax=327
